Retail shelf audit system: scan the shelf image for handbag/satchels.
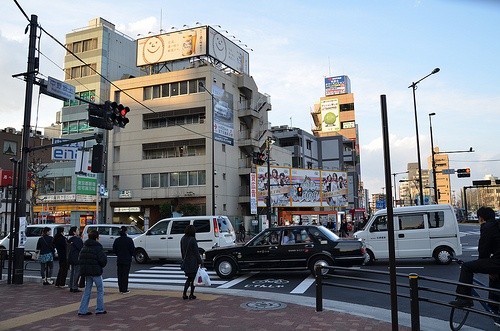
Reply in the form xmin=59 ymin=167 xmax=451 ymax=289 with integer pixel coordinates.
xmin=38 ymin=252 xmax=53 ymax=263
xmin=77 ymin=274 xmax=86 ymax=288
xmin=193 ymin=267 xmax=212 ymax=287
xmin=181 ymin=259 xmax=186 ymax=270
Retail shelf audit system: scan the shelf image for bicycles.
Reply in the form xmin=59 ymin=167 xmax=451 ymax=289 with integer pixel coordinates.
xmin=447 ymin=255 xmax=500 ymax=331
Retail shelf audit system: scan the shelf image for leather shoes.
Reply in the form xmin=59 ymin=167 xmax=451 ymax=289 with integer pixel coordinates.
xmin=448 ymin=299 xmax=474 ymax=308
xmin=70 ymin=288 xmax=74 ymax=292
xmin=72 ymin=288 xmax=82 ymax=292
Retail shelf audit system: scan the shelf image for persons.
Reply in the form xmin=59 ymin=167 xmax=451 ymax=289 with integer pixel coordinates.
xmin=114 ymin=227 xmax=136 ymax=294
xmin=77 ymin=230 xmax=108 ymax=317
xmin=257 ymin=168 xmax=291 ymax=202
xmin=280 ymin=232 xmax=292 ymax=246
xmin=55 ymin=227 xmax=71 ymax=290
xmin=66 ymin=226 xmax=85 ymax=293
xmin=449 ymin=206 xmax=500 ymax=327
xmin=321 ymin=173 xmax=348 ymax=206
xmin=182 ymin=225 xmax=204 ymax=301
xmin=37 ymin=228 xmax=57 ymax=286
xmin=79 ymin=227 xmax=96 ymax=289
xmin=238 ymin=215 xmax=368 ymax=242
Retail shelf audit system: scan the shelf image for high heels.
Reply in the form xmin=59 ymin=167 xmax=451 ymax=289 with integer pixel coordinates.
xmin=183 ymin=294 xmax=188 ymax=300
xmin=189 ymin=295 xmax=196 ymax=299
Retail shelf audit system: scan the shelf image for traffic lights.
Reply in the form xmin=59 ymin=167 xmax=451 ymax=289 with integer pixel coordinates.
xmin=457 ymin=168 xmax=470 ymax=173
xmin=253 ymin=159 xmax=265 ymax=165
xmin=105 ymin=100 xmax=130 ymax=117
xmin=103 ymin=113 xmax=129 ymax=128
xmin=253 ymin=152 xmax=265 ymax=158
xmin=458 ymin=174 xmax=470 ymax=178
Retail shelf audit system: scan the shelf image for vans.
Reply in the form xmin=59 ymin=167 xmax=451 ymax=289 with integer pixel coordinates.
xmin=350 ymin=204 xmax=463 ymax=265
xmin=80 ymin=223 xmax=145 ymax=251
xmin=132 ymin=215 xmax=236 ymax=266
xmin=0 ymin=224 xmax=73 ymax=262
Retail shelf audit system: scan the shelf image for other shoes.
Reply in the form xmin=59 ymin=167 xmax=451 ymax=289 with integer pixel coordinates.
xmin=78 ymin=312 xmax=93 ymax=317
xmin=46 ymin=277 xmax=54 ymax=285
xmin=42 ymin=278 xmax=48 ymax=285
xmin=95 ymin=310 xmax=107 ymax=315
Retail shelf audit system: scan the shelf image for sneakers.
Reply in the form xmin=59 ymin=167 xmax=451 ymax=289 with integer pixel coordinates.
xmin=122 ymin=291 xmax=130 ymax=294
xmin=55 ymin=285 xmax=59 ymax=288
xmin=59 ymin=283 xmax=69 ymax=288
xmin=120 ymin=291 xmax=122 ymax=294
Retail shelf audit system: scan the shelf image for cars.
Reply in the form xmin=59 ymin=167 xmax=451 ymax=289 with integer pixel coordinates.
xmin=202 ymin=225 xmax=370 ymax=279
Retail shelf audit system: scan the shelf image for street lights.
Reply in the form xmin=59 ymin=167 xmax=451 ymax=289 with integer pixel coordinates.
xmin=397 ymin=67 xmax=441 ymax=206
